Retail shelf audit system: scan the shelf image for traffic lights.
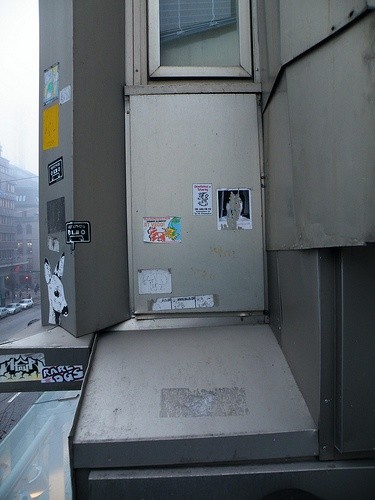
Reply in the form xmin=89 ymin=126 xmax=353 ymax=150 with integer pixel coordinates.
xmin=25 ymin=276 xmax=28 ymax=280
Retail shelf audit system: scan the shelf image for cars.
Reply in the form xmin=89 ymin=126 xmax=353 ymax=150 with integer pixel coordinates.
xmin=0 ymin=307 xmax=8 ymax=319
xmin=6 ymin=303 xmax=22 ymax=315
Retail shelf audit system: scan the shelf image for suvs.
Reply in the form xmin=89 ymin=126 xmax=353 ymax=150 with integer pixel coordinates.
xmin=19 ymin=299 xmax=33 ymax=310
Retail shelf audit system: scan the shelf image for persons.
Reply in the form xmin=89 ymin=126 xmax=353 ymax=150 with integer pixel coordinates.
xmin=8 ymin=282 xmax=40 ymax=299
